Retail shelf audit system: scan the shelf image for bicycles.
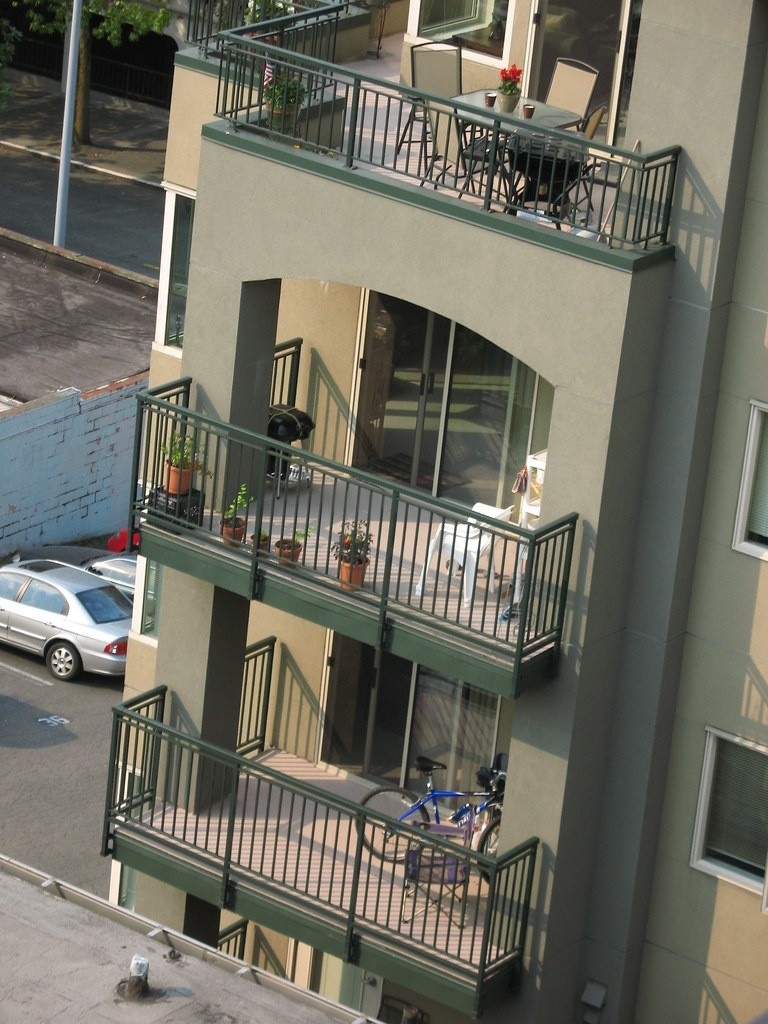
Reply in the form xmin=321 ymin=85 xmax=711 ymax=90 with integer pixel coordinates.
xmin=355 ymin=756 xmax=507 ymax=886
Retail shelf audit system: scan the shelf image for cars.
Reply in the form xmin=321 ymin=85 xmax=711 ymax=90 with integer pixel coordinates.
xmin=0 ymin=559 xmax=154 ymax=681
xmin=107 ymin=524 xmax=140 ymax=555
xmin=11 ymin=546 xmax=158 ymax=619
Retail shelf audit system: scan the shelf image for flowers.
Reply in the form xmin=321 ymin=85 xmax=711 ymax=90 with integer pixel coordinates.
xmin=498 ymin=63 xmax=523 ymax=95
xmin=241 ymin=0 xmax=296 ymax=27
xmin=333 ymin=520 xmax=373 ymax=562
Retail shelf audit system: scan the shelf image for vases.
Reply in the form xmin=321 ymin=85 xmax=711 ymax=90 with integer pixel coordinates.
xmin=336 ymin=554 xmax=370 ymax=593
xmin=497 ymin=92 xmax=521 ymax=113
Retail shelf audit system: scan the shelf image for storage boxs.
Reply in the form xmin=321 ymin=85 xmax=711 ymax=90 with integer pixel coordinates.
xmin=146 ymin=486 xmax=206 ymax=535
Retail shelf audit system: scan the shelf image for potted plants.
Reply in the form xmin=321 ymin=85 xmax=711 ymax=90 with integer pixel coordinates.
xmin=160 ymin=431 xmax=214 ymax=495
xmin=262 ymin=75 xmax=305 ymax=135
xmin=218 ymin=483 xmax=255 ymax=549
xmin=274 ymin=522 xmax=315 ymax=568
xmin=251 ymin=530 xmax=269 ymax=557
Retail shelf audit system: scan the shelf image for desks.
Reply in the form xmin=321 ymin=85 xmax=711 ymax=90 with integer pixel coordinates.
xmin=451 ymin=25 xmax=505 ymax=58
xmin=449 ymin=89 xmax=583 ymax=208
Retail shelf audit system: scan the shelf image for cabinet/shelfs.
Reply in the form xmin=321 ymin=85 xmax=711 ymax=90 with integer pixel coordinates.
xmin=508 ymin=447 xmax=547 ymax=604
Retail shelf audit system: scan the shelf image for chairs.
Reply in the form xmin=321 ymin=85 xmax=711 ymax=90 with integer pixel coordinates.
xmin=415 ymin=502 xmax=516 ymax=609
xmin=400 ymin=802 xmax=475 ymax=930
xmin=394 ymin=41 xmax=624 ymax=225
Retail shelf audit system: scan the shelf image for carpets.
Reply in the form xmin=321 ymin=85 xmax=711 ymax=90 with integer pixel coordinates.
xmin=362 ymin=453 xmax=473 ymax=495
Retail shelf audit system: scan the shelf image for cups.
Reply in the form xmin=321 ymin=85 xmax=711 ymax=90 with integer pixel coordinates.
xmin=485 ymin=93 xmax=497 ymax=107
xmin=523 ymin=104 xmax=535 ymax=118
